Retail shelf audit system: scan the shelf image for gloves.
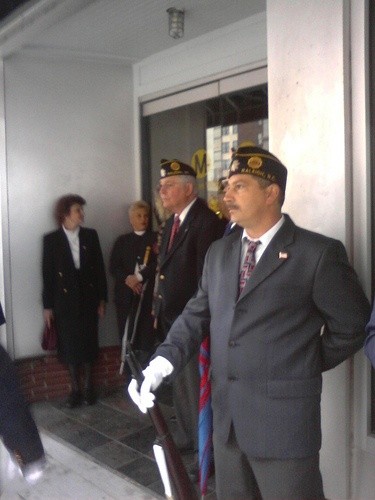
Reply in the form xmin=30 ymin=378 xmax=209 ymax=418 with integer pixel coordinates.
xmin=128 ymin=355 xmax=174 ymax=415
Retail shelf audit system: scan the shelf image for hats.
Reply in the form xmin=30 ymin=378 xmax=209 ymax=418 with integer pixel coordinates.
xmin=218 ymin=177 xmax=228 ymax=192
xmin=228 ymin=146 xmax=288 ymax=189
xmin=160 ymin=158 xmax=196 ymax=179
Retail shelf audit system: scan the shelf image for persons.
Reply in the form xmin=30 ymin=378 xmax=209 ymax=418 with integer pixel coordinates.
xmin=41 ymin=194 xmax=110 ymax=409
xmin=0 ymin=302 xmax=47 ymax=485
xmin=151 ymin=160 xmax=226 ymax=475
xmin=126 ymin=140 xmax=371 ymax=500
xmin=109 ymin=200 xmax=159 ymax=398
xmin=215 ymin=176 xmax=232 ymax=238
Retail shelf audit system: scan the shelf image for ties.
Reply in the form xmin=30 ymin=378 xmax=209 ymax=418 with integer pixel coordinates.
xmin=237 ymin=242 xmax=257 ymax=298
xmin=166 ymin=216 xmax=180 ymax=252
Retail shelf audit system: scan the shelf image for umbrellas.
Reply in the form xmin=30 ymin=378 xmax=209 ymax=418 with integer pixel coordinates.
xmin=196 ymin=335 xmax=214 ymax=499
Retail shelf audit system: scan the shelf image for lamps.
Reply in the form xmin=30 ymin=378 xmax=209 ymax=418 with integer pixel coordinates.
xmin=166 ymin=6 xmax=185 ymax=40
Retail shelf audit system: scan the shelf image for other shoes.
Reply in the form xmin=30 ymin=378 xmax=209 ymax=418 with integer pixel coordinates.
xmin=83 ymin=392 xmax=94 ymax=405
xmin=64 ymin=393 xmax=81 ymax=408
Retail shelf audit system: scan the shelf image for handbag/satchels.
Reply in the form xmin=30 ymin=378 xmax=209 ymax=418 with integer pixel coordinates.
xmin=42 ymin=319 xmax=57 ymax=349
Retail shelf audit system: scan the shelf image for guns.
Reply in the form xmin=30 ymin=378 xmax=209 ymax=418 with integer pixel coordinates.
xmin=124 ymin=341 xmax=199 ymax=500
